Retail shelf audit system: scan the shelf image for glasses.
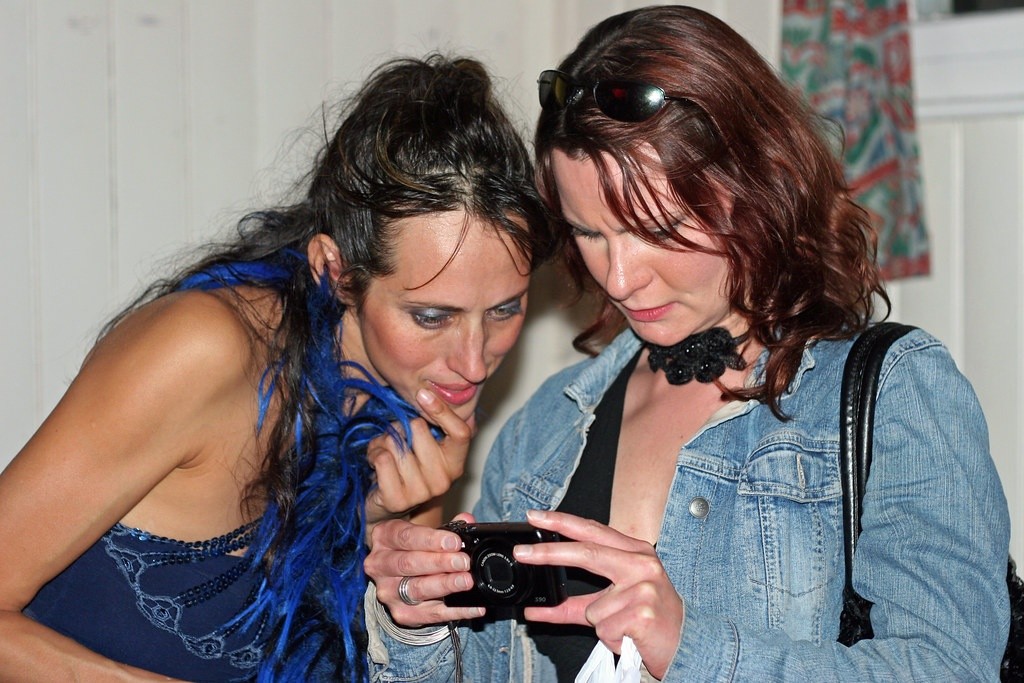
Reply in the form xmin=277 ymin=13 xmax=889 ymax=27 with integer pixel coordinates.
xmin=538 ymin=70 xmax=718 ymax=137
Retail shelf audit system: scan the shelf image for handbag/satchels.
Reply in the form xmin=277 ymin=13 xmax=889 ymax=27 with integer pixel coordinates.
xmin=836 ymin=322 xmax=1024 ymax=683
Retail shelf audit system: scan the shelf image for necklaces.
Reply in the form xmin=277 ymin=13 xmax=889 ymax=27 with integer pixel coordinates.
xmin=647 ymin=324 xmax=753 ymax=385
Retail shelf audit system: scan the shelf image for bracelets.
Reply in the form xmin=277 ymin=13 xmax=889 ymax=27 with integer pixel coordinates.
xmin=373 ymin=587 xmax=454 ymax=645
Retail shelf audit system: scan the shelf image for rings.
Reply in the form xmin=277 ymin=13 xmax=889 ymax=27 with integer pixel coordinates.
xmin=398 ymin=577 xmax=421 ymax=605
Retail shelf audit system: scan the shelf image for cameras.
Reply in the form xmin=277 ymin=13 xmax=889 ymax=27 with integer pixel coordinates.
xmin=437 ymin=520 xmax=568 ymax=628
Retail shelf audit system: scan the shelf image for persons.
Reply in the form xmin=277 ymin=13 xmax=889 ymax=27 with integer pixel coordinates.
xmin=363 ymin=4 xmax=1010 ymax=683
xmin=0 ymin=54 xmax=555 ymax=683
